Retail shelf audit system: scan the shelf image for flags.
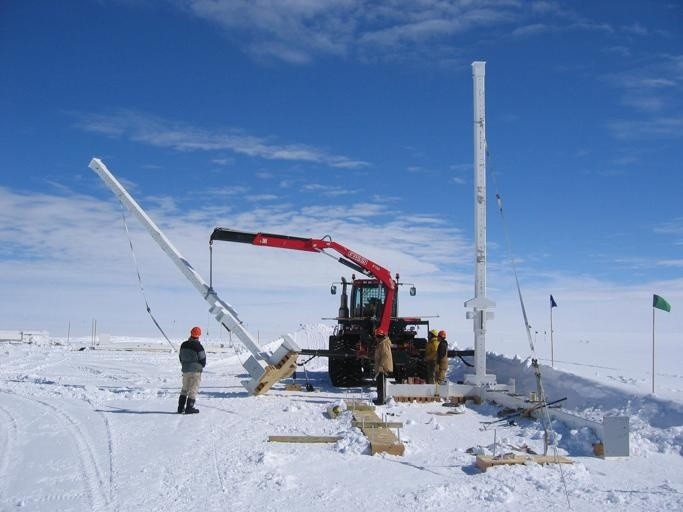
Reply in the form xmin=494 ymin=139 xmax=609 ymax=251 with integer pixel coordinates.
xmin=551 ymin=295 xmax=557 ymax=307
xmin=653 ymin=294 xmax=671 ymax=312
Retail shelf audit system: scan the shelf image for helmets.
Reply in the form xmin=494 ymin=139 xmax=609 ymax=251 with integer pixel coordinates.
xmin=374 ymin=329 xmax=383 ymax=337
xmin=430 ymin=328 xmax=439 ymax=336
xmin=190 ymin=327 xmax=201 ymax=337
xmin=438 ymin=330 xmax=446 ymax=338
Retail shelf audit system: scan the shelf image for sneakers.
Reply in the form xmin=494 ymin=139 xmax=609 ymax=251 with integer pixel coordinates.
xmin=373 ymin=398 xmax=385 ymax=405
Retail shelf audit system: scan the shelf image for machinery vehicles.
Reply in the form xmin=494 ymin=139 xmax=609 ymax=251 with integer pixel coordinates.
xmin=209 ymin=227 xmax=429 ymax=388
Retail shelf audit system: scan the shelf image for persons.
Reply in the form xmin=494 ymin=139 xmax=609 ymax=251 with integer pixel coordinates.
xmin=424 ymin=329 xmax=439 ymax=383
xmin=373 ymin=328 xmax=394 ymax=405
xmin=178 ymin=327 xmax=206 ymax=414
xmin=436 ymin=331 xmax=448 ymax=384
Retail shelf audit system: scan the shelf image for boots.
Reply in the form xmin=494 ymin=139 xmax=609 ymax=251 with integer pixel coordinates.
xmin=178 ymin=394 xmax=186 ymax=413
xmin=186 ymin=397 xmax=199 ymax=414
